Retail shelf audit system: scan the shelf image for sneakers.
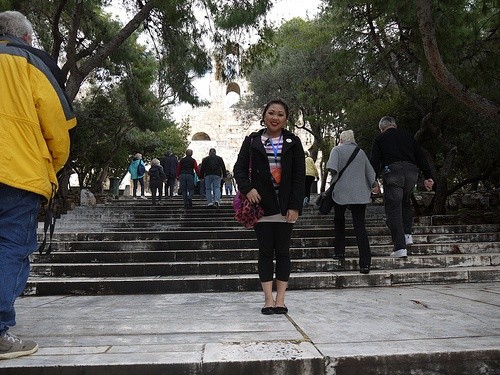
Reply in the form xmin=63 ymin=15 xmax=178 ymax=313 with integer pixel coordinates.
xmin=0 ymin=332 xmax=39 ymax=359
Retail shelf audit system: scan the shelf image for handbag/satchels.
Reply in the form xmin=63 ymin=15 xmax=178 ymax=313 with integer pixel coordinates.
xmin=315 ymin=183 xmax=333 ymax=214
xmin=157 ymin=165 xmax=168 ymax=184
xmin=137 ymin=160 xmax=145 ymax=176
xmin=233 ymin=190 xmax=263 ymax=228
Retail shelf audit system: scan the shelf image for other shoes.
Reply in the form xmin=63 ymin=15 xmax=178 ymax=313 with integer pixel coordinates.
xmin=141 ymin=196 xmax=147 ymax=199
xmin=275 ymin=302 xmax=288 ymax=314
xmin=389 ymin=248 xmax=408 ymax=258
xmin=405 ymin=234 xmax=413 ymax=245
xmin=359 ymin=264 xmax=370 ymax=273
xmin=133 ymin=197 xmax=138 ymax=200
xmin=215 ymin=201 xmax=221 ymax=206
xmin=303 ymin=197 xmax=308 ymax=207
xmin=158 ymin=201 xmax=164 ymax=206
xmin=261 ymin=300 xmax=275 ymax=314
xmin=332 ymin=254 xmax=346 ymax=261
xmin=208 ymin=203 xmax=213 ymax=206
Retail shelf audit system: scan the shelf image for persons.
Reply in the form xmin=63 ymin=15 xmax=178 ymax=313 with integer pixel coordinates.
xmin=147 ymin=158 xmax=168 ymax=205
xmin=129 ymin=153 xmax=147 ymax=200
xmin=372 ymin=116 xmax=434 ymax=258
xmin=0 ymin=10 xmax=77 ymax=360
xmin=304 ymin=150 xmax=319 ymax=203
xmin=233 ymin=98 xmax=305 ymax=315
xmin=177 ymin=149 xmax=200 ymax=208
xmin=326 ymin=130 xmax=375 ymax=273
xmin=161 ymin=150 xmax=178 ymax=197
xmin=177 ymin=155 xmax=238 ymax=199
xmin=200 ymin=148 xmax=226 ymax=206
